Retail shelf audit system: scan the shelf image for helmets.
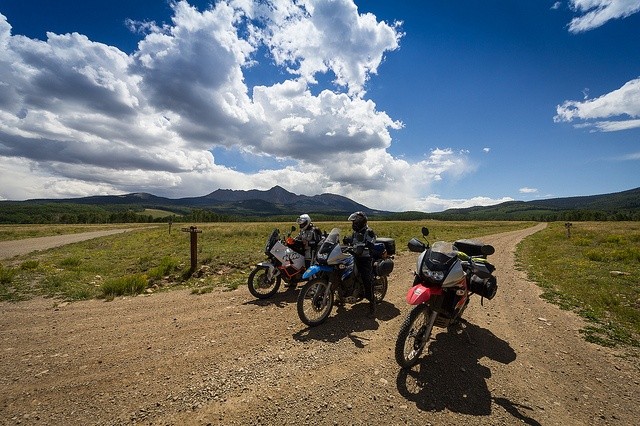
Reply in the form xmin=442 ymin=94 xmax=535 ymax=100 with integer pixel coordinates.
xmin=296 ymin=214 xmax=311 ymax=230
xmin=348 ymin=211 xmax=368 ymax=232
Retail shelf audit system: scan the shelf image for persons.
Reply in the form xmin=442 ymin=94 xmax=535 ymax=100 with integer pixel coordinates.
xmin=295 ymin=214 xmax=315 ymax=297
xmin=344 ymin=212 xmax=377 ymax=317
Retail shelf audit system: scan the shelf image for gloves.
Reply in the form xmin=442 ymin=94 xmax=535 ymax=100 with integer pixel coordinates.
xmin=357 ymin=245 xmax=365 ymax=251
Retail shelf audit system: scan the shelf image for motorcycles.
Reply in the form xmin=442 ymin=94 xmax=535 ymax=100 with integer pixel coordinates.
xmin=297 ymin=227 xmax=395 ymax=327
xmin=248 ymin=226 xmax=340 ymax=299
xmin=395 ymin=226 xmax=497 ymax=368
